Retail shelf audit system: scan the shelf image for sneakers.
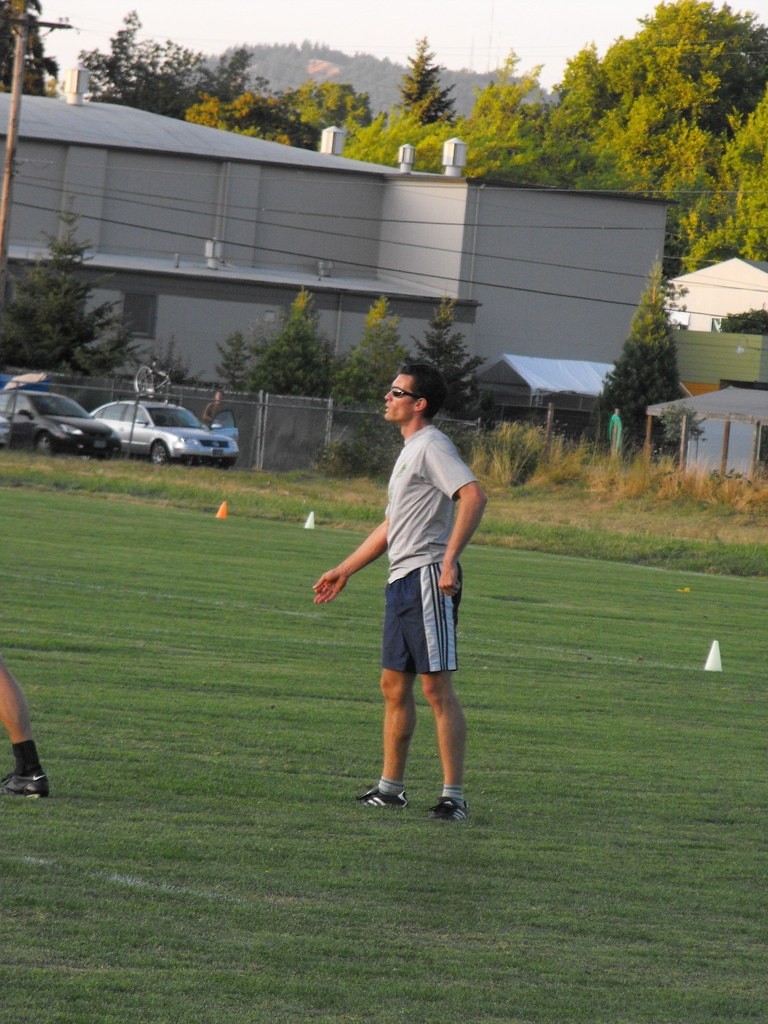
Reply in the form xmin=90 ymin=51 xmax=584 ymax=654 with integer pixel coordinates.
xmin=427 ymin=797 xmax=470 ymax=822
xmin=0 ymin=772 xmax=49 ymax=797
xmin=355 ymin=787 xmax=408 ymax=808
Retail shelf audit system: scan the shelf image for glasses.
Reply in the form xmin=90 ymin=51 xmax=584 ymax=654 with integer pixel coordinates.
xmin=392 ymin=387 xmax=422 ymax=398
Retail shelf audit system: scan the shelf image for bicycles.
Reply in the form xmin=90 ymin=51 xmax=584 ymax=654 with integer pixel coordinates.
xmin=131 ymin=355 xmax=173 ymax=403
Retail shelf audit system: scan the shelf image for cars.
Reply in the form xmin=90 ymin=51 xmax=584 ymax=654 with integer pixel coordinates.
xmin=89 ymin=400 xmax=240 ymax=468
xmin=0 ymin=388 xmax=122 ymax=460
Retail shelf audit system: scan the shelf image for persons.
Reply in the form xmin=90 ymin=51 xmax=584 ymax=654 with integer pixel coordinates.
xmin=313 ymin=363 xmax=488 ymax=823
xmin=0 ymin=661 xmax=51 ymax=801
xmin=202 ymin=389 xmax=227 ymax=427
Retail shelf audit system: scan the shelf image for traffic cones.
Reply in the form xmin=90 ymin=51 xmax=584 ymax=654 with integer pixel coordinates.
xmin=704 ymin=639 xmax=723 ymax=672
xmin=214 ymin=500 xmax=228 ymax=519
xmin=303 ymin=511 xmax=315 ymax=529
